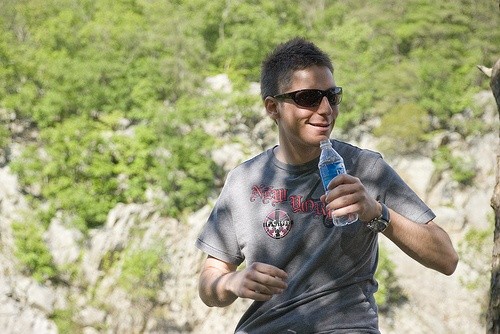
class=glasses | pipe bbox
[274,87,342,108]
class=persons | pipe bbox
[196,37,459,333]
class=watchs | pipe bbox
[367,202,391,234]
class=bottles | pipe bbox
[318,139,358,227]
